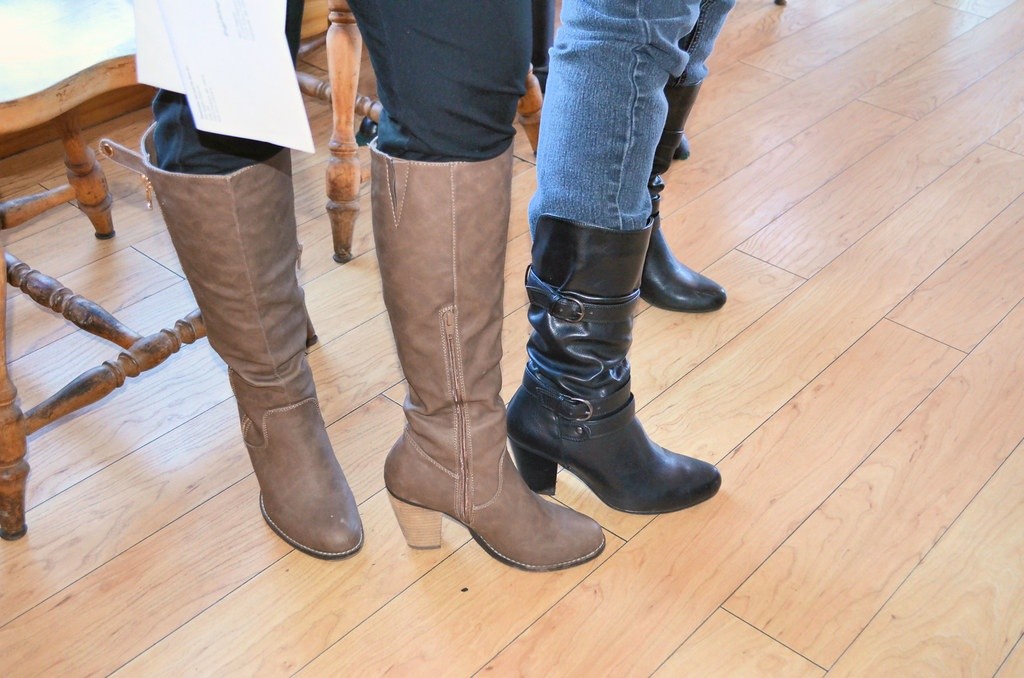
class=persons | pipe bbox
[100,0,604,572]
[507,0,736,515]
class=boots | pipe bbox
[504,213,720,515]
[368,136,608,573]
[97,122,364,561]
[639,78,728,313]
